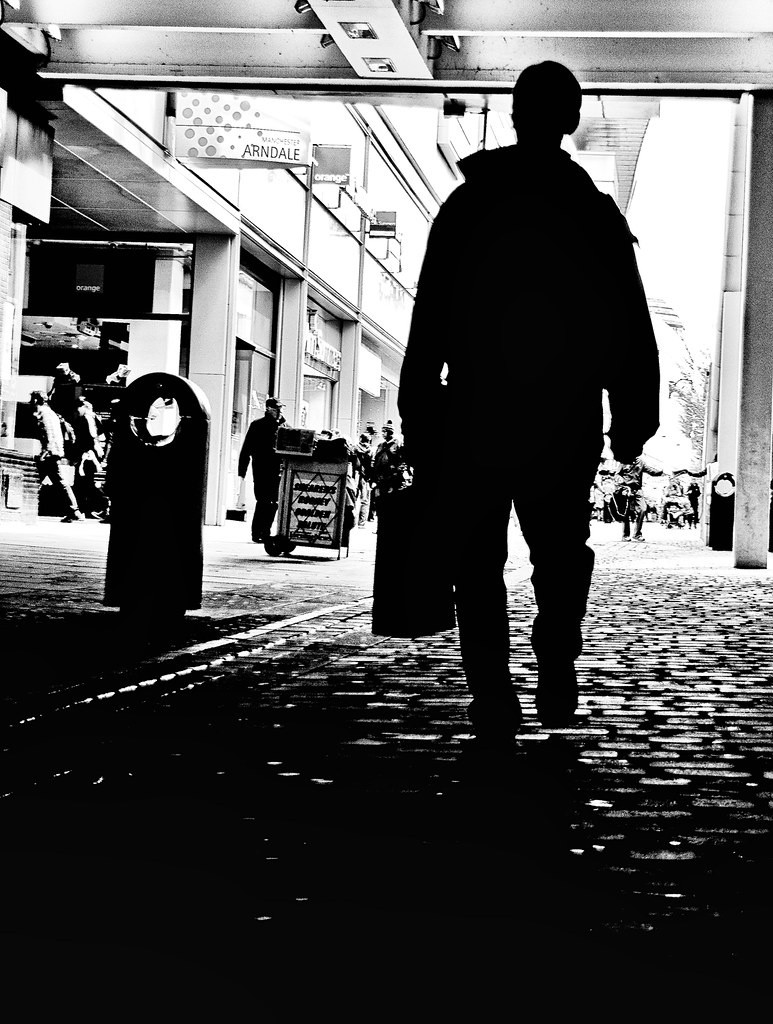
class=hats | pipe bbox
[381,420,393,433]
[265,398,282,411]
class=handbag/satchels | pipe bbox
[372,480,456,639]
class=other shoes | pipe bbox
[634,534,645,541]
[534,656,577,726]
[466,699,516,749]
[623,536,631,541]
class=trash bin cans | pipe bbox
[708,472,736,552]
[98,370,211,612]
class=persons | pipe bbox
[613,455,662,542]
[61,395,120,524]
[317,419,403,529]
[240,397,293,541]
[396,61,662,758]
[688,478,701,529]
[26,391,85,522]
